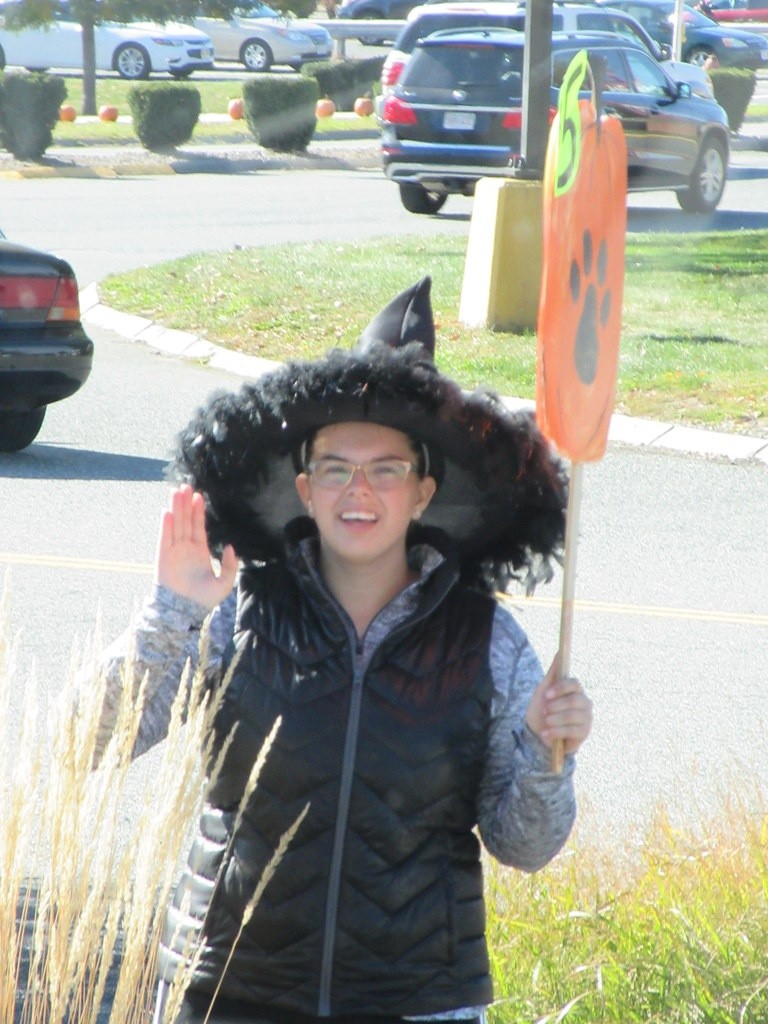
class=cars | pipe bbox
[174,0,334,71]
[0,0,215,80]
[0,231,94,451]
[337,0,460,44]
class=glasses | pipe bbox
[307,460,419,489]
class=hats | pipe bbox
[160,276,568,609]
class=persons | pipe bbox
[58,419,593,1024]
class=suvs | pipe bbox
[338,0,768,213]
